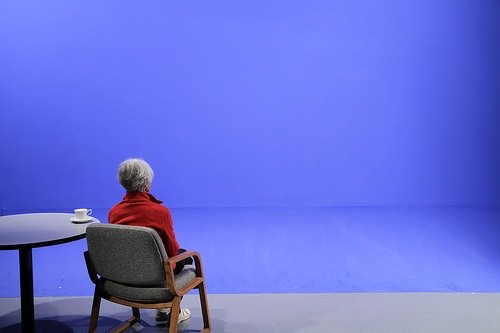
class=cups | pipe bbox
[74,208,92,220]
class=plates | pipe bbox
[70,217,92,222]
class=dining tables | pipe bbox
[0,213,101,333]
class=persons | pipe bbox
[108,159,192,326]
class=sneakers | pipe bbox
[156,305,191,328]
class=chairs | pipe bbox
[83,223,213,333]
